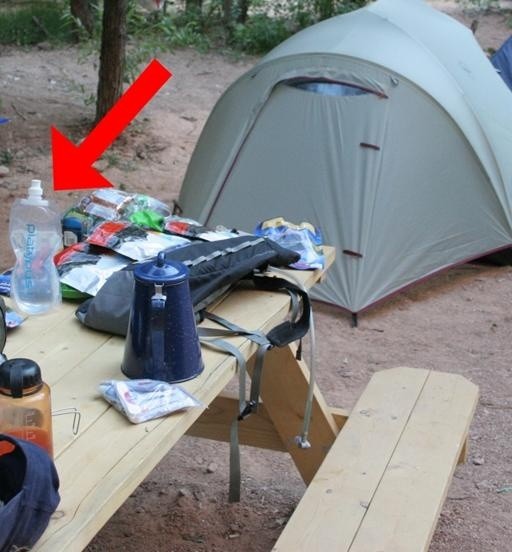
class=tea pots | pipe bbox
[120,252,206,381]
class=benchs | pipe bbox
[275,362,481,551]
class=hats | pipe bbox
[1,433,61,552]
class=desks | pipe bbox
[1,228,348,552]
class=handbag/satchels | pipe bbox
[76,235,302,338]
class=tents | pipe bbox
[176,0,512,327]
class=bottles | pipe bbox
[1,356,53,461]
